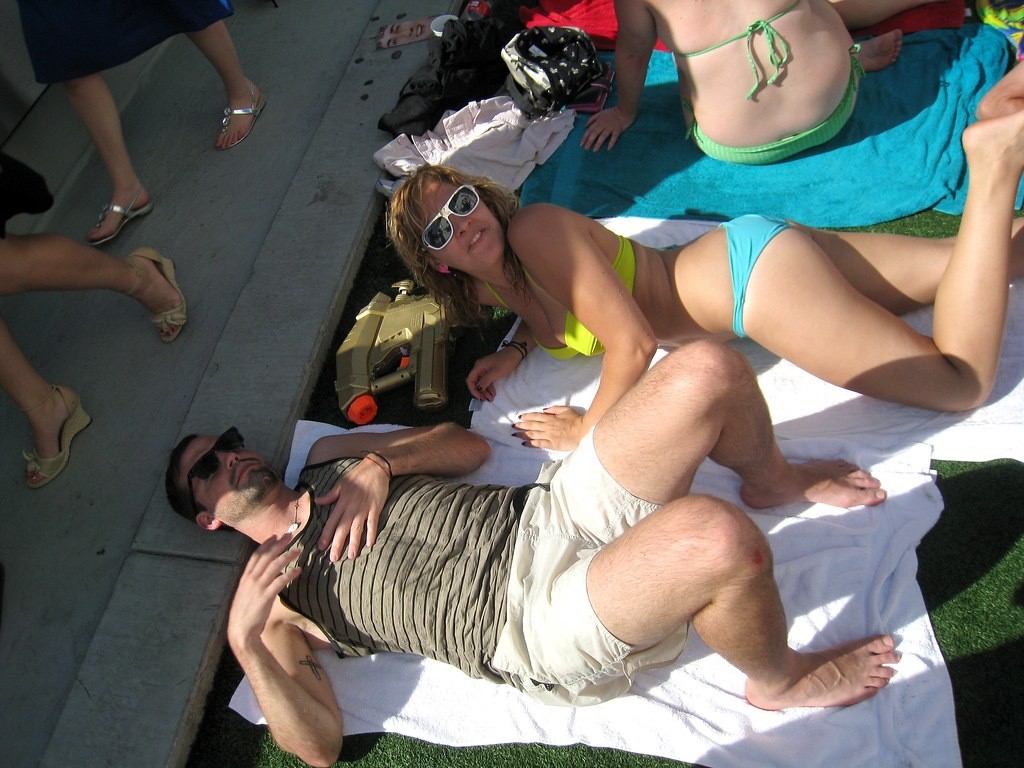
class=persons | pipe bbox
[580,0,902,164]
[976,60,1024,119]
[17,0,267,244]
[164,340,902,768]
[829,0,934,29]
[389,110,1024,451]
[1,148,187,487]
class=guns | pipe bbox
[333,277,479,426]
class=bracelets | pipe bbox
[364,451,394,479]
[501,340,527,360]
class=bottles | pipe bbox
[460,0,495,22]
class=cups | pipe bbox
[431,15,458,37]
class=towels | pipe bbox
[225,416,962,768]
[469,22,1024,232]
[512,0,965,52]
[464,215,1024,465]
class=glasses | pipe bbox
[187,426,244,515]
[422,184,479,252]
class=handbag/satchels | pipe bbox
[500,25,604,121]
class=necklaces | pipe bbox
[287,493,299,533]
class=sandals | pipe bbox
[84,184,154,246]
[20,385,91,489]
[124,248,187,342]
[215,81,267,150]
